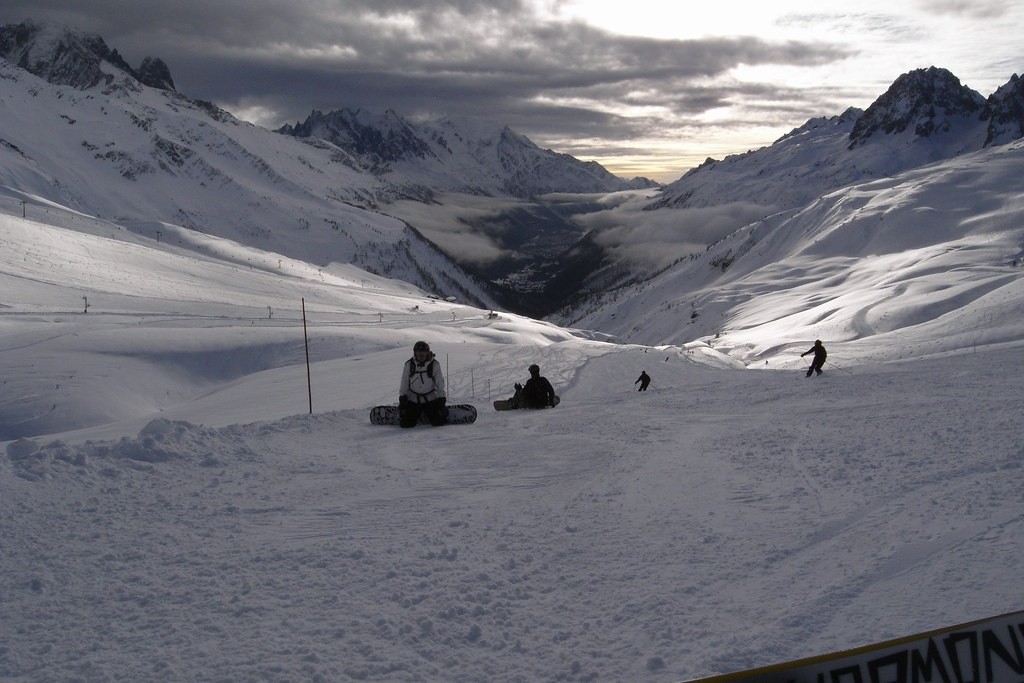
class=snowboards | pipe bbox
[369,404,478,426]
[493,395,561,411]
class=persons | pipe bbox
[508,364,555,409]
[397,341,449,428]
[635,370,650,390]
[801,340,827,378]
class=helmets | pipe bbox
[414,341,431,351]
[529,365,540,373]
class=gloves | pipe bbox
[549,400,555,408]
[438,399,451,418]
[514,383,522,393]
[399,399,410,420]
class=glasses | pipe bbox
[415,352,430,357]
